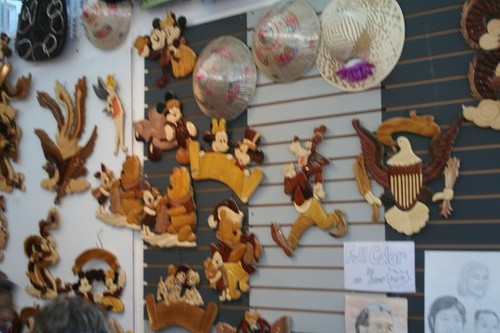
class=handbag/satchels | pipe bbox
[15,0,68,61]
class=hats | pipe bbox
[81,0,133,50]
[193,34,257,122]
[252,0,321,82]
[315,0,406,91]
